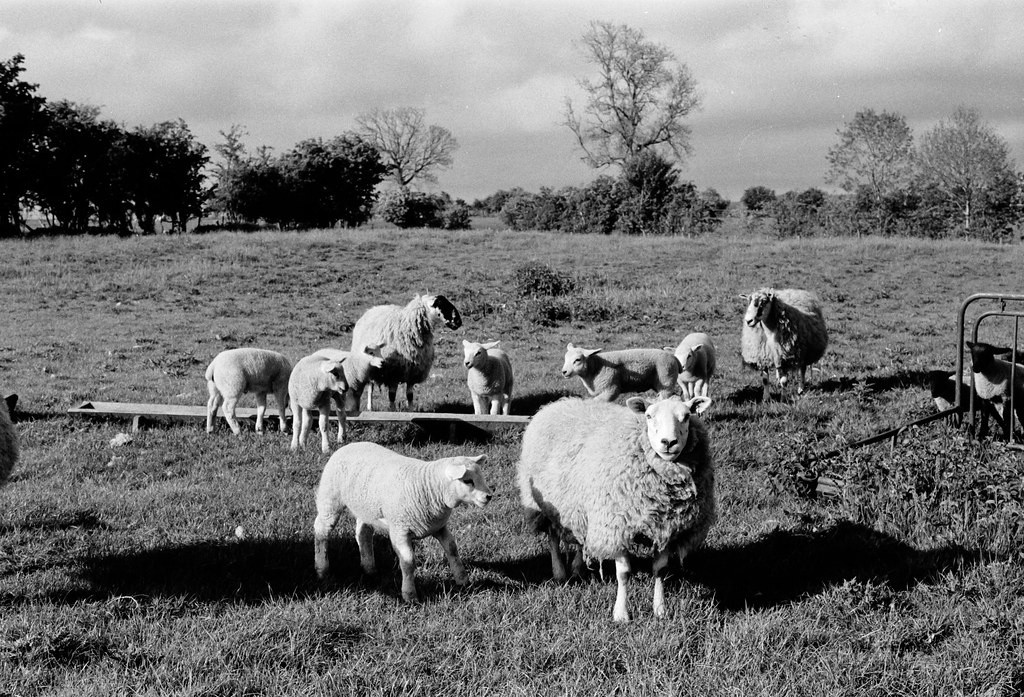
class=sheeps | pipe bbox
[205,348,292,437]
[0,394,20,485]
[518,390,717,623]
[463,340,514,415]
[288,342,388,455]
[314,441,493,604]
[562,343,682,404]
[739,287,828,401]
[663,333,716,396]
[351,287,463,411]
